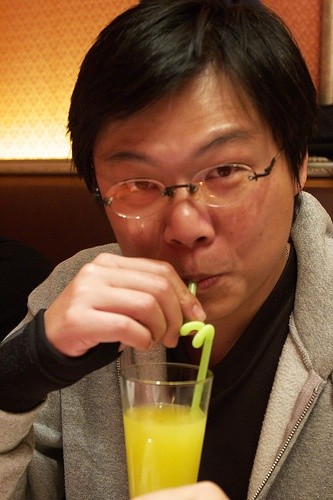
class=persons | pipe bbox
[0,0,333,500]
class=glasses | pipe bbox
[96,144,285,219]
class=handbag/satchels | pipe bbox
[0,235,54,329]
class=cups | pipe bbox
[119,363,214,500]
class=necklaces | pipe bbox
[284,248,289,261]
